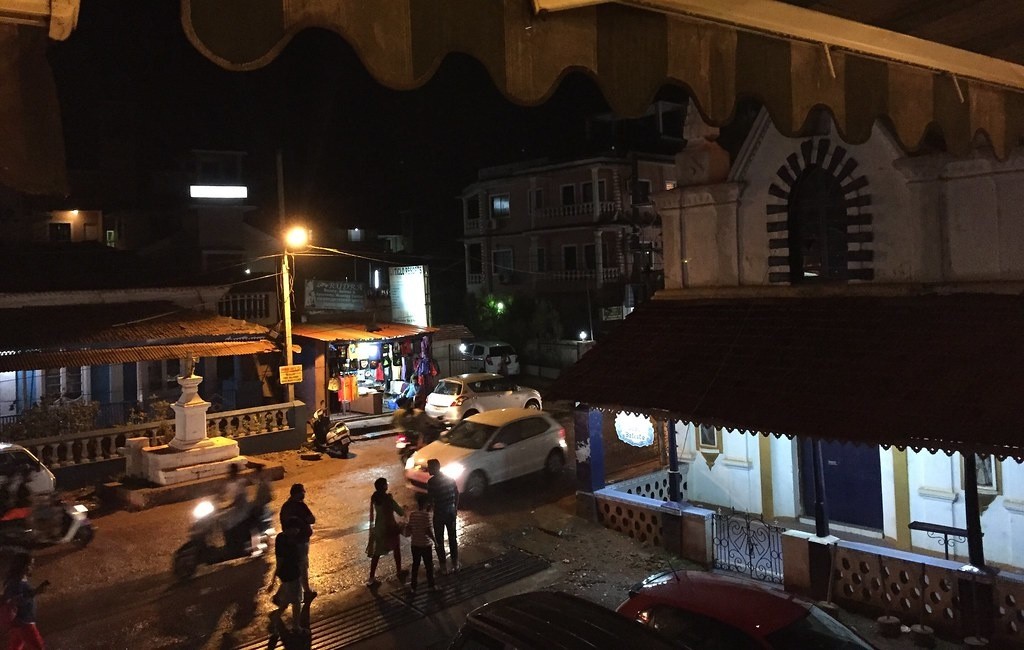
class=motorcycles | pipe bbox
[396,413,451,464]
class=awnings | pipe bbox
[175,0,1024,160]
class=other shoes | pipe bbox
[302,590,317,604]
[438,568,449,575]
[451,561,464,575]
[366,577,381,586]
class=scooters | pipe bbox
[307,402,351,458]
[0,493,94,559]
[170,500,273,572]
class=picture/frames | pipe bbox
[699,423,717,448]
[963,453,997,491]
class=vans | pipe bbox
[0,443,56,497]
[447,590,692,650]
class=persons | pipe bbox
[391,397,444,443]
[485,354,511,379]
[408,492,437,593]
[0,466,48,539]
[211,462,273,546]
[427,459,459,576]
[366,478,409,587]
[271,484,317,636]
[400,376,423,401]
[0,552,51,650]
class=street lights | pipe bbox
[279,221,309,403]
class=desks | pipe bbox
[341,392,382,415]
[906,521,985,561]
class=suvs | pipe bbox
[616,570,878,650]
[403,408,570,503]
[425,373,543,429]
[462,342,520,375]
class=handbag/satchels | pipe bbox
[396,513,411,538]
